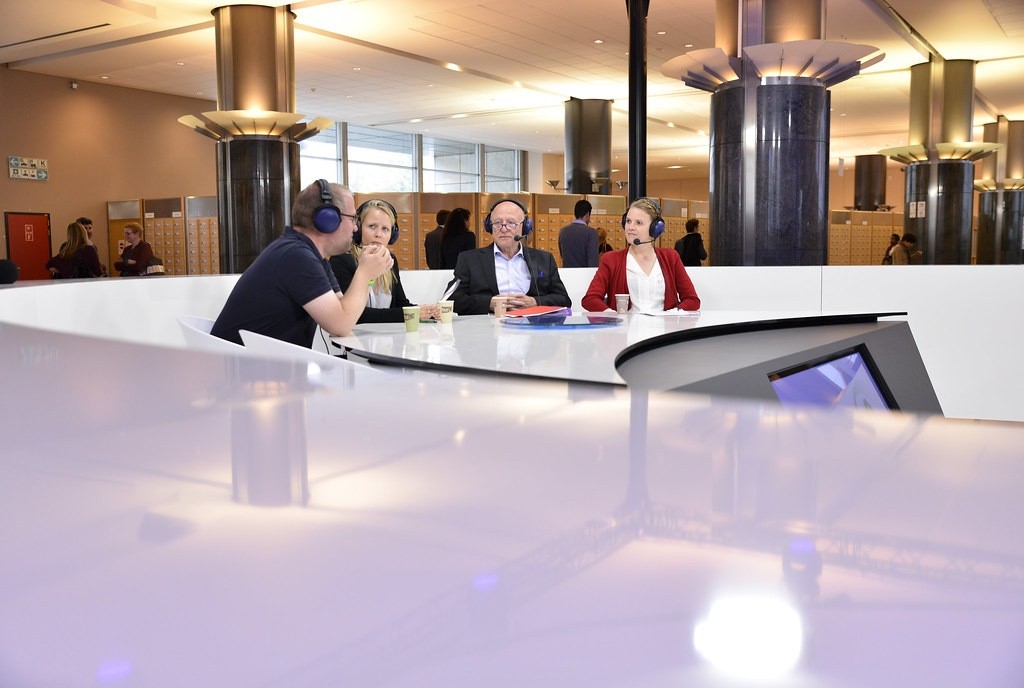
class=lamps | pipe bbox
[973,177,1024,192]
[545,178,629,191]
[878,141,1003,165]
[844,206,854,210]
[177,109,334,141]
[878,205,897,211]
[662,40,885,93]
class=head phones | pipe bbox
[485,198,533,236]
[353,199,400,246]
[621,197,666,239]
[312,179,342,233]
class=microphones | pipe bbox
[514,235,527,241]
[634,238,657,245]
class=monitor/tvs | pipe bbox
[768,344,893,413]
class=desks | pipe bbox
[329,310,907,391]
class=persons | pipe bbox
[674,218,707,266]
[46,218,103,279]
[211,178,394,350]
[558,200,613,269]
[581,199,701,313]
[882,233,922,265]
[114,222,154,277]
[330,199,436,323]
[443,199,572,315]
[425,208,475,270]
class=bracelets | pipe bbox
[368,280,374,285]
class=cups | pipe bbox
[615,294,630,314]
[492,297,507,318]
[437,300,454,323]
[402,306,421,332]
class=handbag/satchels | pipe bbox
[140,257,164,276]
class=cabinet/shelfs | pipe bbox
[828,211,903,265]
[143,197,221,275]
[352,192,714,270]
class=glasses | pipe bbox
[490,222,523,230]
[341,214,359,224]
[124,232,133,235]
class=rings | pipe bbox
[428,309,430,314]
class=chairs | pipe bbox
[179,316,370,369]
